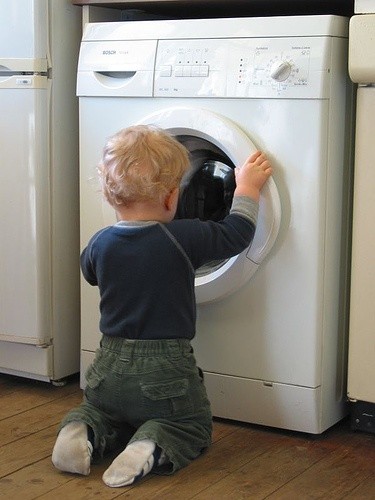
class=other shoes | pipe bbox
[61,449,62,449]
[52,422,94,475]
[102,439,161,485]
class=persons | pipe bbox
[52,126,273,488]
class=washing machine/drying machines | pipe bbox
[76,13,344,434]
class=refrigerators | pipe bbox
[0,0,83,387]
